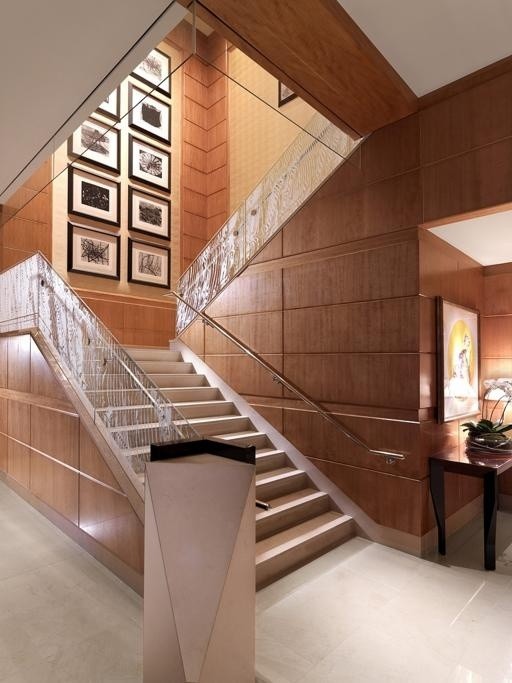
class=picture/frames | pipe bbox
[127,46,171,288]
[278,80,297,107]
[67,78,120,280]
[433,294,482,425]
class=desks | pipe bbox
[428,439,512,571]
